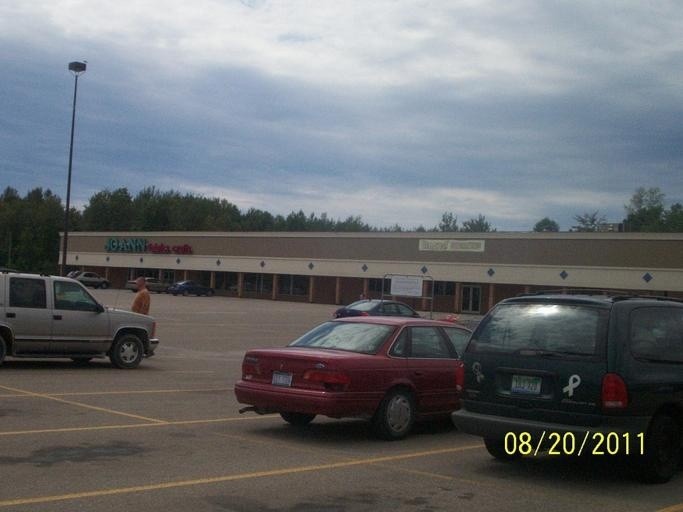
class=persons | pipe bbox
[131,276,155,358]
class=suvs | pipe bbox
[450,288,683,485]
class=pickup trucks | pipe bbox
[0,267,160,370]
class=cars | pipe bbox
[234,316,475,443]
[331,299,421,318]
[66,270,215,297]
